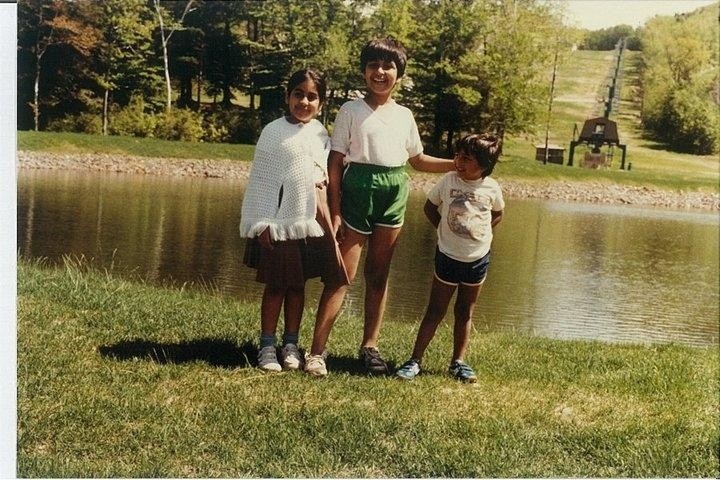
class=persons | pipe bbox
[394,131,507,383]
[237,65,350,373]
[303,35,457,376]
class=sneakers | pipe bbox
[359,346,389,374]
[280,342,303,371]
[303,347,328,376]
[256,343,284,372]
[396,359,422,379]
[449,360,477,384]
